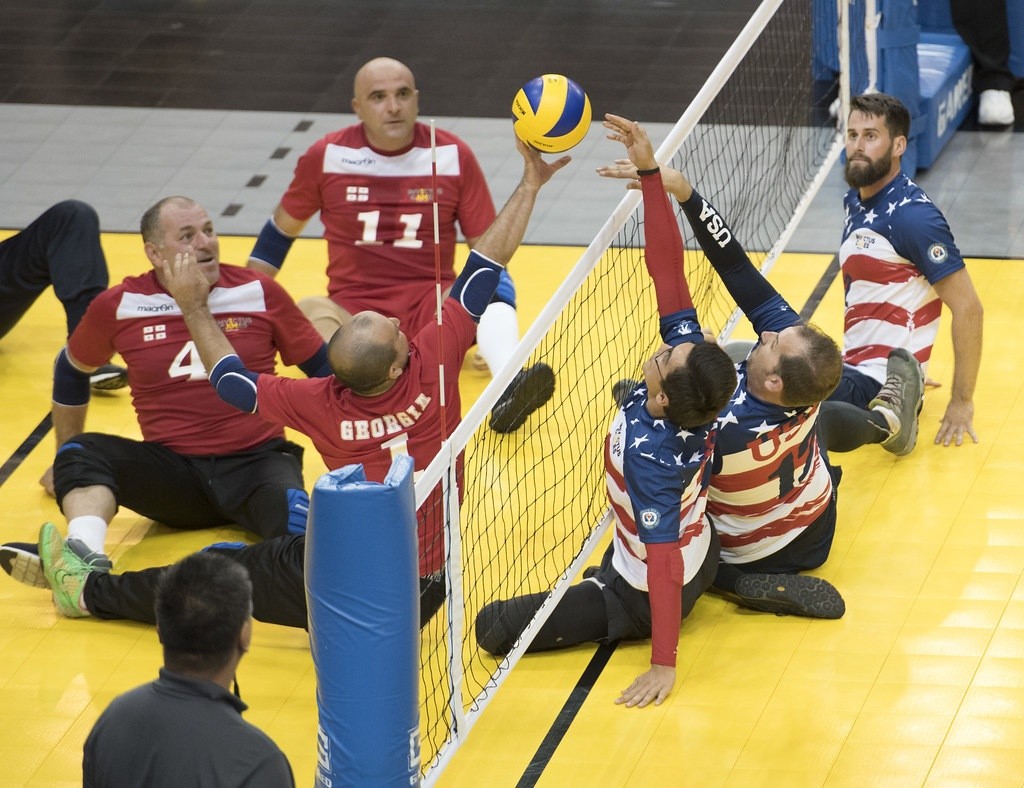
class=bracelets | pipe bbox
[636,167,661,176]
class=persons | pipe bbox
[828,0,1014,124]
[82,551,296,788]
[475,94,984,707]
[0,56,572,632]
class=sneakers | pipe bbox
[612,378,640,409]
[735,572,846,620]
[866,348,924,456]
[0,538,112,589]
[489,362,555,433]
[583,567,601,578]
[89,361,129,389]
[39,522,114,618]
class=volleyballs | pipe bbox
[510,72,592,154]
